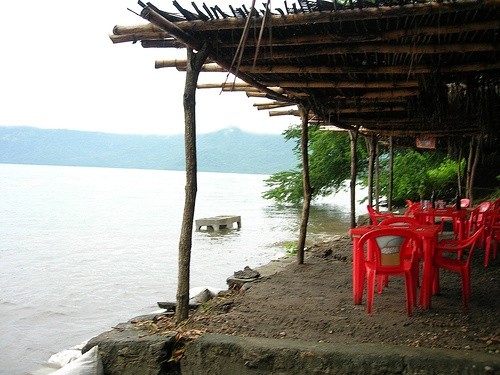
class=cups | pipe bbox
[420,199,429,211]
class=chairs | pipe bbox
[349,198,499,318]
